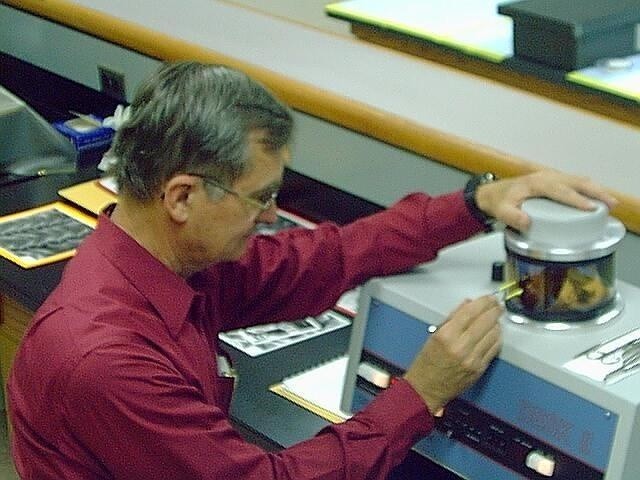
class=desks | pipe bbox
[1,86,418,480]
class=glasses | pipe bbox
[202,173,278,213]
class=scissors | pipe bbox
[587,337,640,365]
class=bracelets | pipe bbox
[463,171,496,226]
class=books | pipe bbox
[279,352,353,420]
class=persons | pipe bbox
[7,59,621,479]
